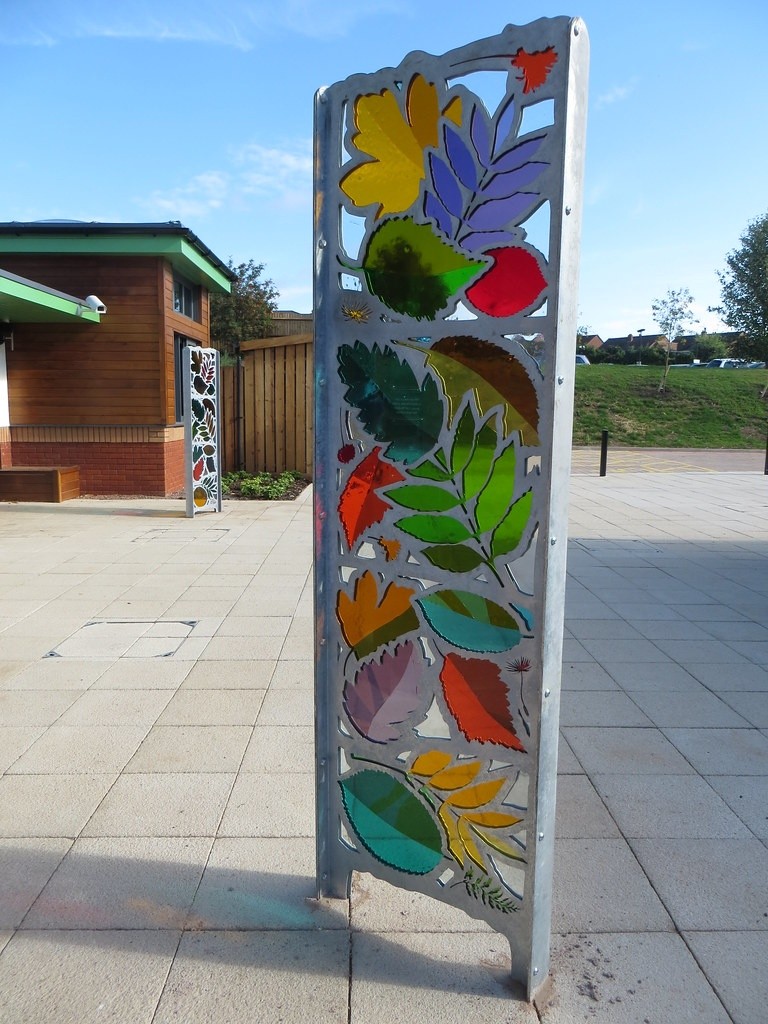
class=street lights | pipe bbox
[637,328,646,366]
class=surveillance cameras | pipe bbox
[86,295,107,313]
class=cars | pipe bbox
[747,361,767,369]
[576,354,591,366]
[690,363,709,368]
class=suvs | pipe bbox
[706,358,748,371]
